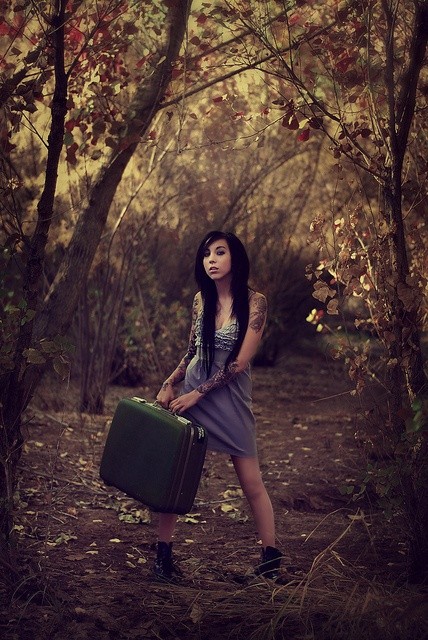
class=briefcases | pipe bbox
[101,398,207,515]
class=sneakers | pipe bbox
[258,546,283,582]
[152,540,181,582]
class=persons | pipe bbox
[156,230,283,584]
